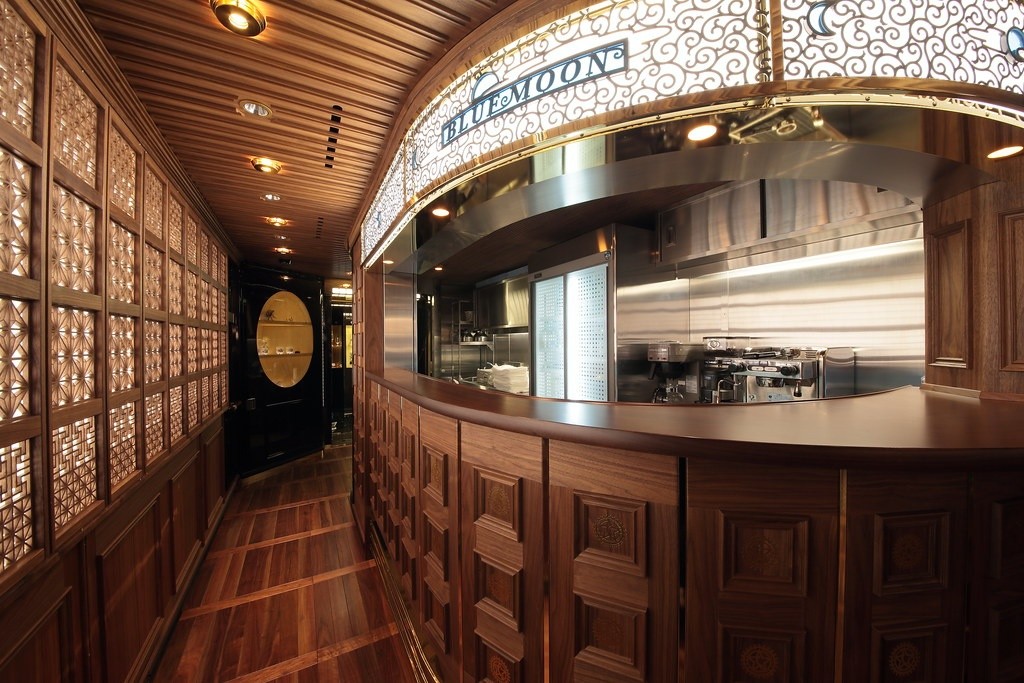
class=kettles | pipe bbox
[461,330,475,342]
[474,328,488,341]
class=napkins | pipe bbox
[493,364,530,393]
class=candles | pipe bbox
[293,368,297,381]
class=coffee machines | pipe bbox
[647,340,707,403]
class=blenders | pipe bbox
[700,333,750,403]
